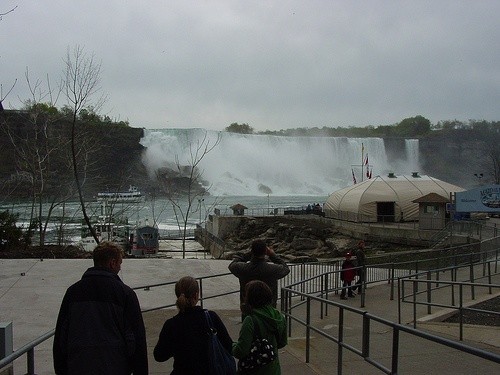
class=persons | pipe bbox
[53,242,148,375]
[352,240,367,294]
[233,280,289,375]
[227,240,290,321]
[153,277,236,375]
[340,252,356,300]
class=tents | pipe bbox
[323,172,469,222]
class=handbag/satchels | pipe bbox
[238,315,276,373]
[203,311,237,375]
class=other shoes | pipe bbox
[351,290,356,295]
[341,296,348,300]
[348,293,355,297]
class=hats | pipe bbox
[249,239,266,250]
[245,280,271,301]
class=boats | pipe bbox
[79,221,137,253]
[96,184,145,204]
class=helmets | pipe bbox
[346,253,351,256]
